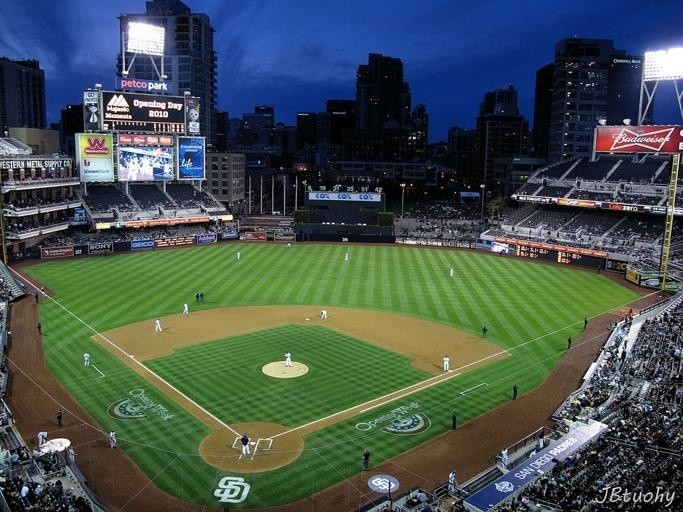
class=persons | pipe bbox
[1,155,681,510]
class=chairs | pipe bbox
[44,177,237,245]
[0,261,103,510]
[394,151,683,510]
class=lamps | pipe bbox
[638,47,682,124]
[119,16,168,82]
